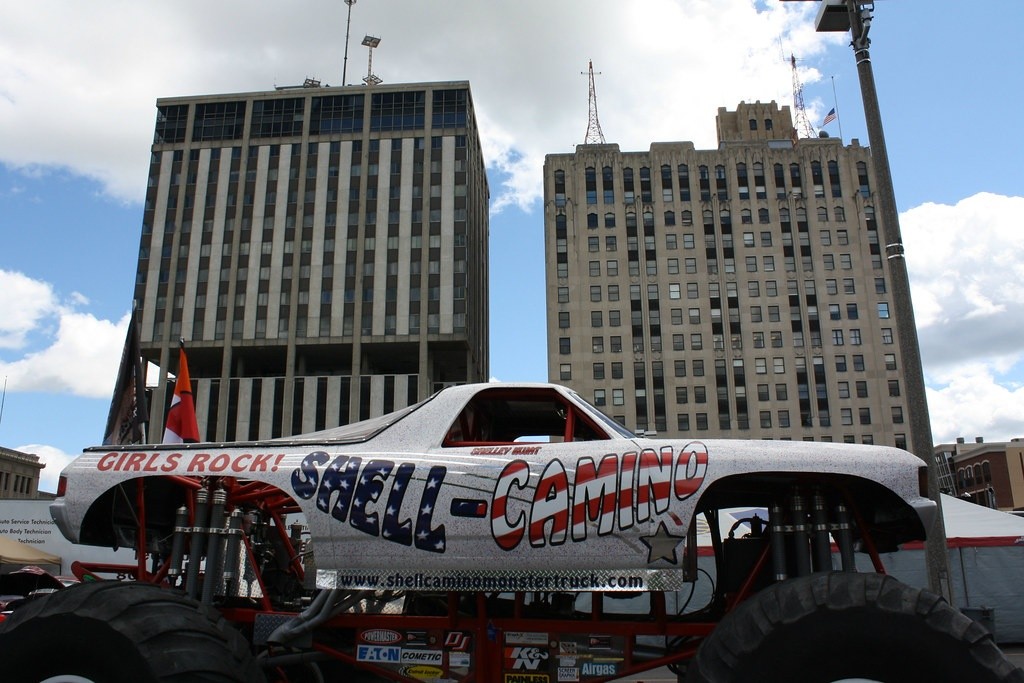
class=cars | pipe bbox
[0,566,79,619]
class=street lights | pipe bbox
[814,0,951,598]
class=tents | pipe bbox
[487,489,1024,656]
[0,534,62,576]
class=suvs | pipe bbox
[1,380,1023,683]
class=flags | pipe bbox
[823,108,835,126]
[102,306,149,445]
[162,348,200,444]
